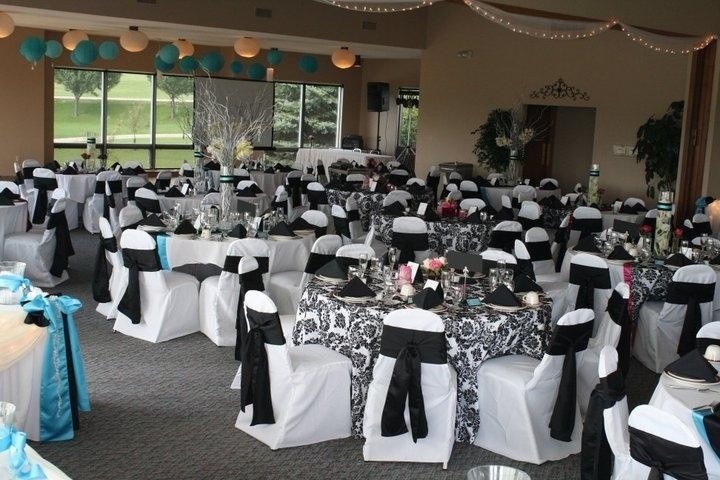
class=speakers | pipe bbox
[366,81,391,112]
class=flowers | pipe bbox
[206,137,254,165]
[495,124,535,149]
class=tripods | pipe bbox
[396,108,416,165]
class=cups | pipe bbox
[0,260,26,306]
[523,291,539,304]
[0,400,19,479]
[466,464,533,480]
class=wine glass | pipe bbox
[154,197,286,241]
[588,226,718,268]
[346,242,517,312]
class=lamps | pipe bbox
[0,12,16,39]
[62,28,89,51]
[171,38,195,59]
[396,91,419,109]
[331,48,356,68]
[120,26,149,52]
[234,36,262,57]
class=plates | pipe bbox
[660,360,720,388]
[314,270,374,305]
[269,228,316,240]
[482,299,530,312]
